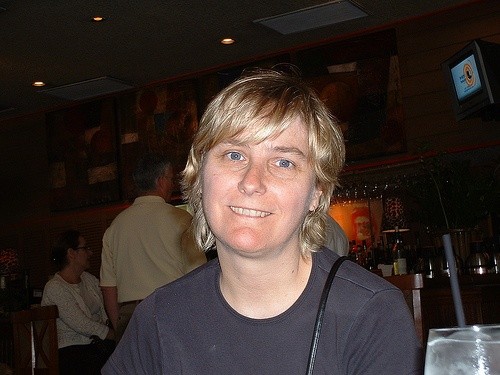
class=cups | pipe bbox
[424,325,500,375]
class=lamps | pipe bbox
[380,194,413,276]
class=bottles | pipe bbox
[343,225,443,276]
[455,241,500,273]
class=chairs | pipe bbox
[9,306,61,375]
[383,271,424,355]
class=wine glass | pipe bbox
[327,172,427,205]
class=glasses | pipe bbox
[76,245,91,251]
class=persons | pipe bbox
[99,154,208,340]
[322,213,349,258]
[100,70,423,374]
[43,231,120,375]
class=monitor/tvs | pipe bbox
[444,39,500,118]
